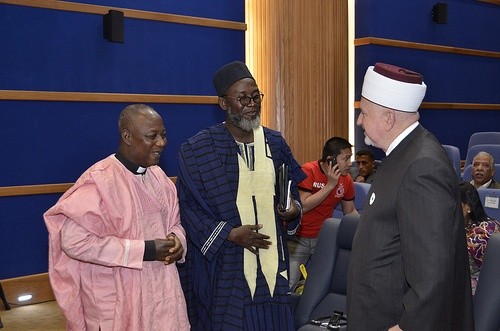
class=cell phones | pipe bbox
[327,155,337,171]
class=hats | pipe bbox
[361,63,428,113]
[213,62,256,95]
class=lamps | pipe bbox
[432,3,448,24]
[103,9,125,43]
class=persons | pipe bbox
[284,137,361,289]
[60,104,188,331]
[178,75,303,331]
[346,63,464,331]
[459,182,500,295]
[352,149,378,184]
[469,151,500,189]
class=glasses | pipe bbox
[223,92,264,105]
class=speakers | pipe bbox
[103,9,124,44]
[432,3,448,24]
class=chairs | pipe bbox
[289,132,500,331]
